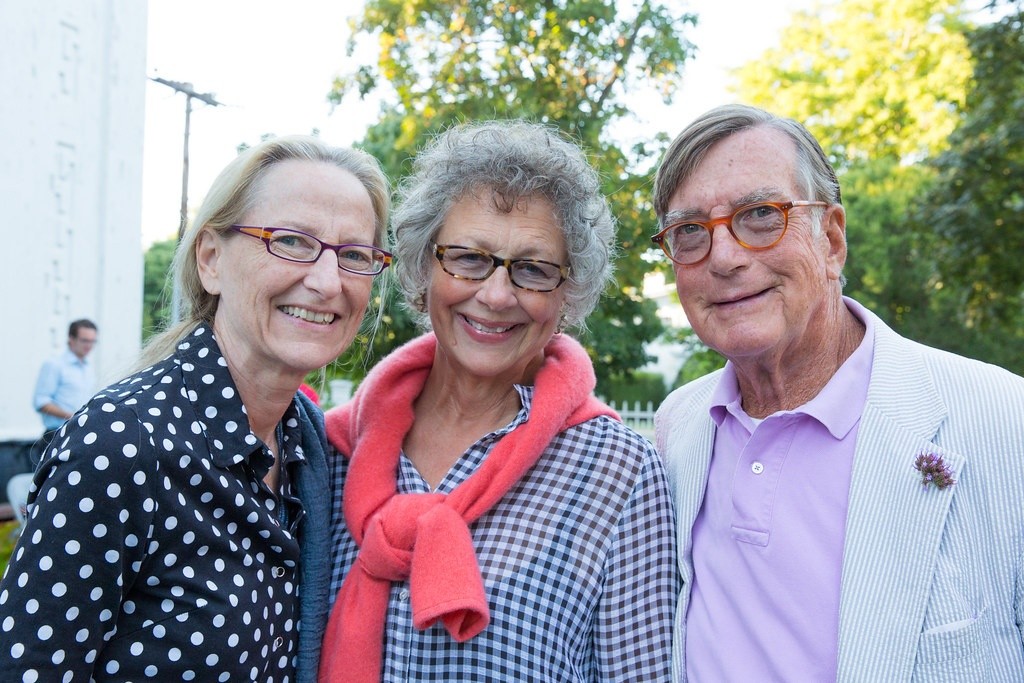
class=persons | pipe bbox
[32,319,99,458]
[319,121,683,683]
[650,103,1023,683]
[0,136,394,683]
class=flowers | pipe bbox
[912,451,956,488]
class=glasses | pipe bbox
[229,223,393,275]
[428,240,571,293]
[651,200,828,266]
